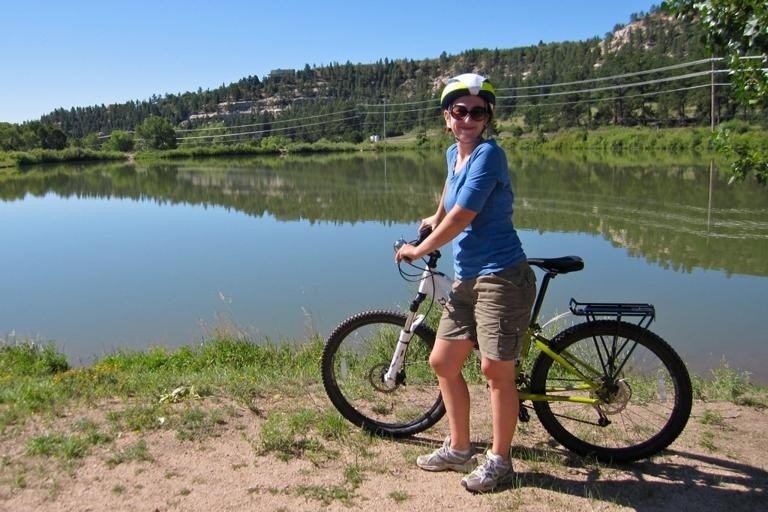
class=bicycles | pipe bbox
[320,225,694,464]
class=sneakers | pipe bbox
[461,448,515,492]
[416,435,478,474]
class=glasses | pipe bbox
[449,105,490,121]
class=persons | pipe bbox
[394,72,536,493]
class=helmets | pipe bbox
[440,73,496,108]
